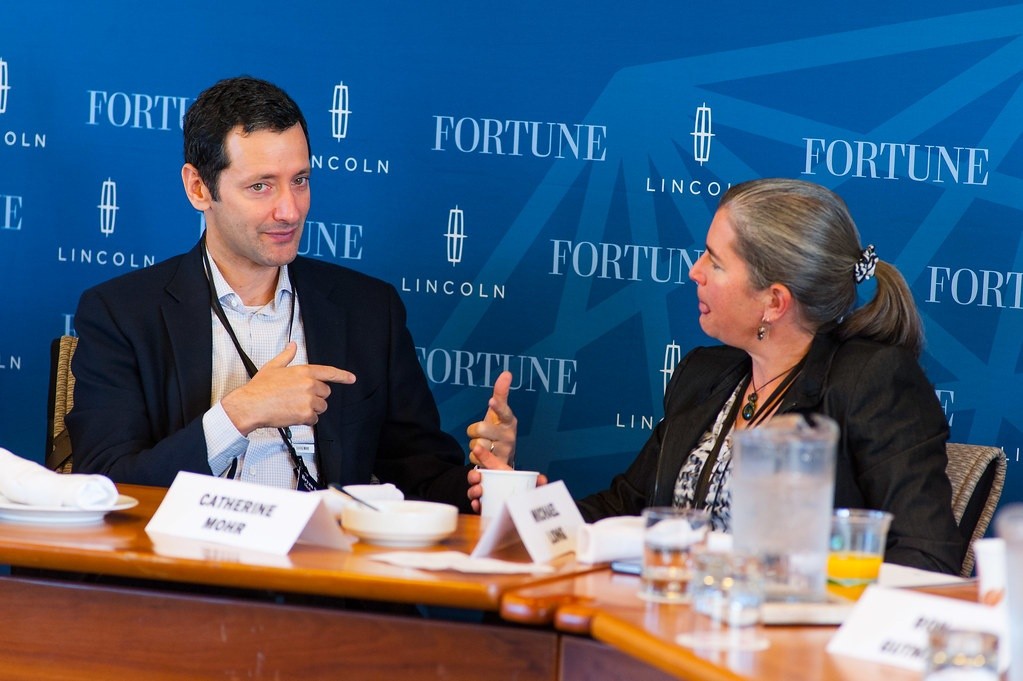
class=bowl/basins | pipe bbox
[343,500,458,549]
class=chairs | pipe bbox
[945,444,1008,577]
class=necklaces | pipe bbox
[742,363,797,421]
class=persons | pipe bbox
[469,177,963,577]
[62,72,516,514]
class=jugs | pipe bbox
[732,413,840,609]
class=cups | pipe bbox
[972,538,1005,607]
[996,504,1023,681]
[641,507,715,604]
[827,509,894,603]
[477,468,539,545]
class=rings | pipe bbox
[490,441,494,452]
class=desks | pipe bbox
[0,478,1008,681]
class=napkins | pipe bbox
[0,446,118,507]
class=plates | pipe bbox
[597,515,644,528]
[0,494,140,525]
[310,484,405,520]
